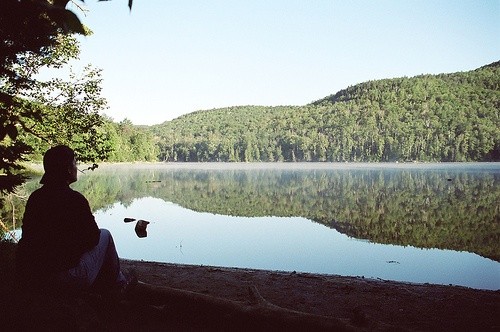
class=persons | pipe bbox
[16,145,127,296]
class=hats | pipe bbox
[39,145,76,185]
